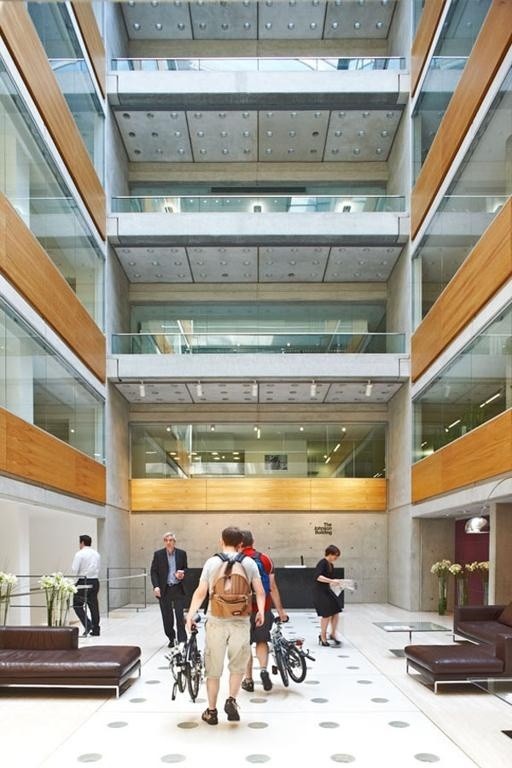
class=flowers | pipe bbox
[464,559,494,593]
[38,572,78,627]
[0,570,17,624]
[448,563,467,606]
[431,558,452,615]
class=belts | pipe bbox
[167,582,182,587]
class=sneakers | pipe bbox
[202,671,272,725]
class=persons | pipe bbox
[238,529,287,692]
[185,525,266,725]
[311,544,343,646]
[64,534,100,636]
[150,532,188,647]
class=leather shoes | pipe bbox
[168,641,174,647]
[83,625,94,635]
[90,632,100,636]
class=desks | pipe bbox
[372,620,452,658]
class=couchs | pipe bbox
[0,625,143,699]
[402,603,512,693]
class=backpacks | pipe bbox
[211,553,252,618]
[252,552,269,603]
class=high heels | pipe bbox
[319,635,340,646]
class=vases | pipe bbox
[482,582,488,606]
[437,577,449,616]
[456,578,468,607]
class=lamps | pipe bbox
[462,473,511,535]
[138,378,375,398]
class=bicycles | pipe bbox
[166,614,205,704]
[268,613,316,688]
